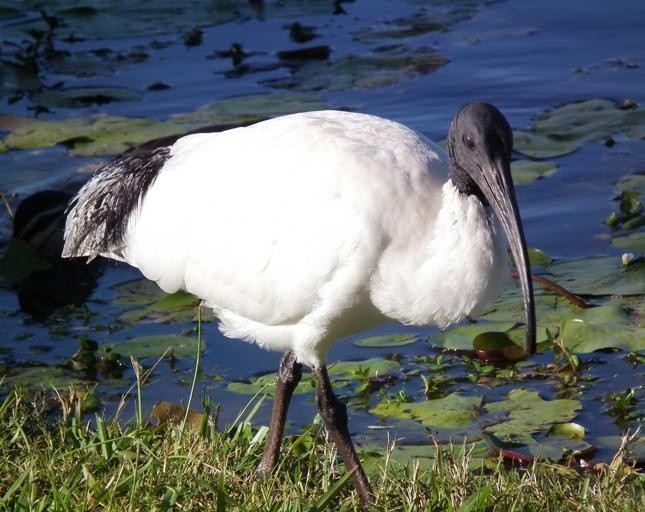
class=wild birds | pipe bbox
[10,100,537,507]
